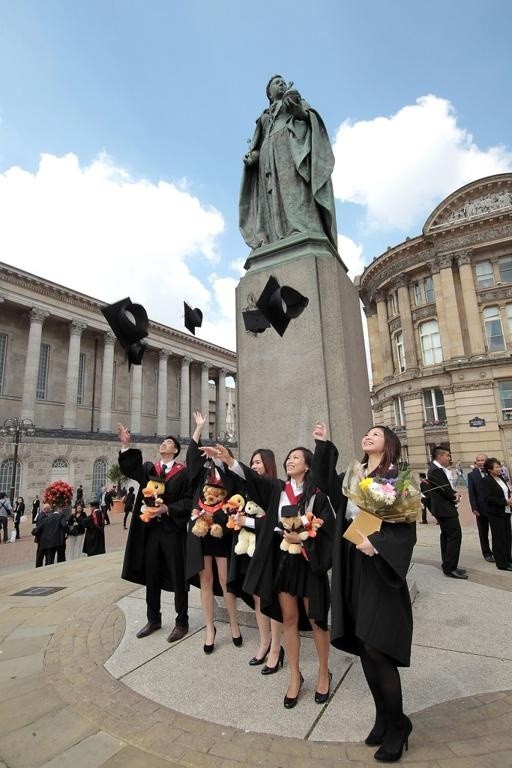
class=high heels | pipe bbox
[248,637,274,666]
[372,713,414,762]
[260,643,285,675]
[230,623,243,647]
[365,713,386,748]
[202,626,217,655]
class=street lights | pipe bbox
[1,417,35,511]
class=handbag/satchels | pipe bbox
[6,509,12,516]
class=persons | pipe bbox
[419,446,512,579]
[115,420,189,643]
[198,446,285,675]
[236,72,339,251]
[213,442,336,709]
[311,418,418,763]
[185,411,243,654]
[0,483,136,568]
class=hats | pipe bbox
[182,300,204,336]
[88,500,100,507]
[100,297,152,348]
[241,308,271,336]
[124,344,147,372]
[255,275,310,339]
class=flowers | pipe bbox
[43,480,75,509]
[357,467,412,519]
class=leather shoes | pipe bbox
[136,622,162,638]
[484,555,495,563]
[498,562,512,571]
[445,570,469,580]
[167,623,189,642]
[455,567,466,575]
[314,672,333,704]
[283,676,304,709]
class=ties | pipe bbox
[159,464,167,480]
[482,470,487,477]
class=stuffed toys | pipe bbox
[274,505,309,555]
[222,493,246,531]
[190,481,228,540]
[138,476,167,525]
[234,500,266,559]
